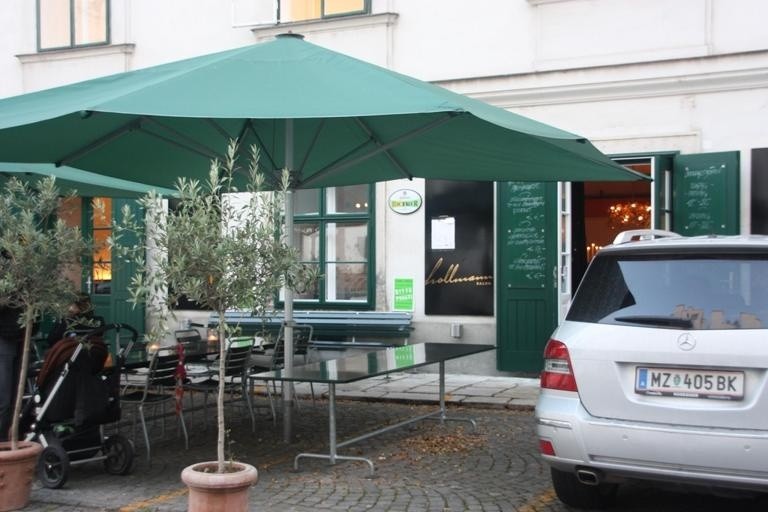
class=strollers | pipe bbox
[23,323,137,490]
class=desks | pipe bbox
[248,341,497,480]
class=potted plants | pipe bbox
[107,136,329,512]
[0,173,108,512]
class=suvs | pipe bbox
[535,225,767,501]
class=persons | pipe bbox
[0,247,33,441]
[45,288,105,351]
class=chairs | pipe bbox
[209,311,415,379]
[120,324,318,467]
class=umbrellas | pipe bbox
[1,26,658,399]
[1,161,194,231]
